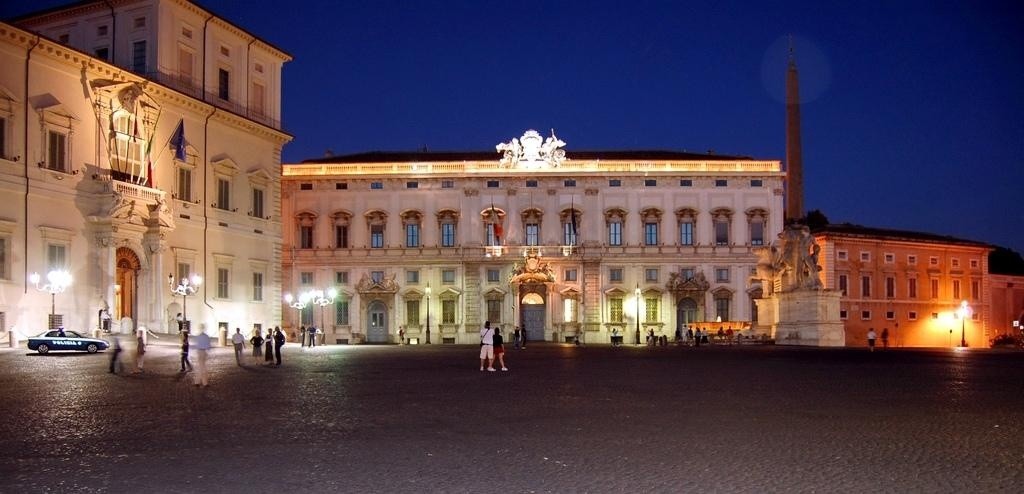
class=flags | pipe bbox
[146,147,154,187]
[571,202,576,234]
[490,205,504,236]
[166,116,186,162]
[132,104,144,141]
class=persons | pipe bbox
[249,329,264,367]
[231,327,245,366]
[190,323,211,388]
[100,309,110,331]
[490,326,508,371]
[398,326,404,345]
[271,326,285,366]
[106,337,122,374]
[880,326,889,347]
[513,326,523,350]
[264,328,275,367]
[300,321,305,347]
[646,328,653,345]
[672,323,734,349]
[574,335,579,345]
[866,326,877,351]
[175,312,182,335]
[135,336,146,373]
[478,319,496,371]
[306,322,315,346]
[180,331,192,372]
[894,321,903,347]
[520,324,526,349]
[611,326,619,345]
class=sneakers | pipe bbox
[487,367,497,371]
[480,367,484,371]
[502,368,508,371]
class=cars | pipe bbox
[26,326,110,354]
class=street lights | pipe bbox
[955,299,973,346]
[634,282,641,343]
[424,281,432,343]
[167,272,203,347]
[309,287,337,345]
[31,268,74,329]
[285,292,312,342]
